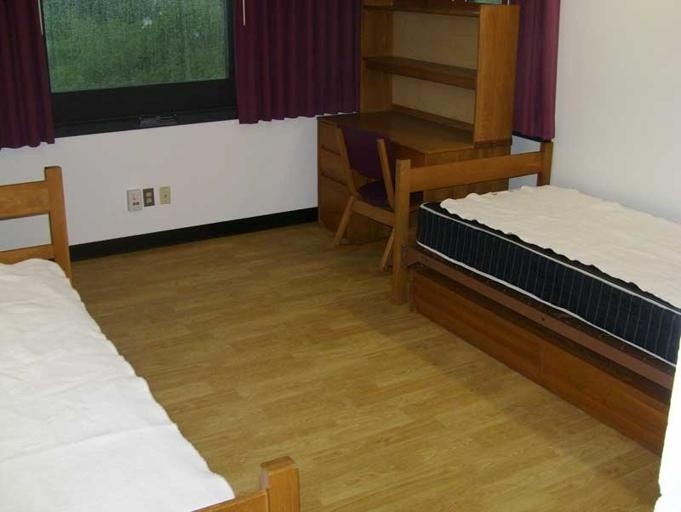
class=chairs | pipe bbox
[327,123,420,272]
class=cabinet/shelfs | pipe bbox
[316,0,520,274]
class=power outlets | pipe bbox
[144,186,154,208]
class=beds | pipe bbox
[0,166,303,512]
[388,140,681,458]
